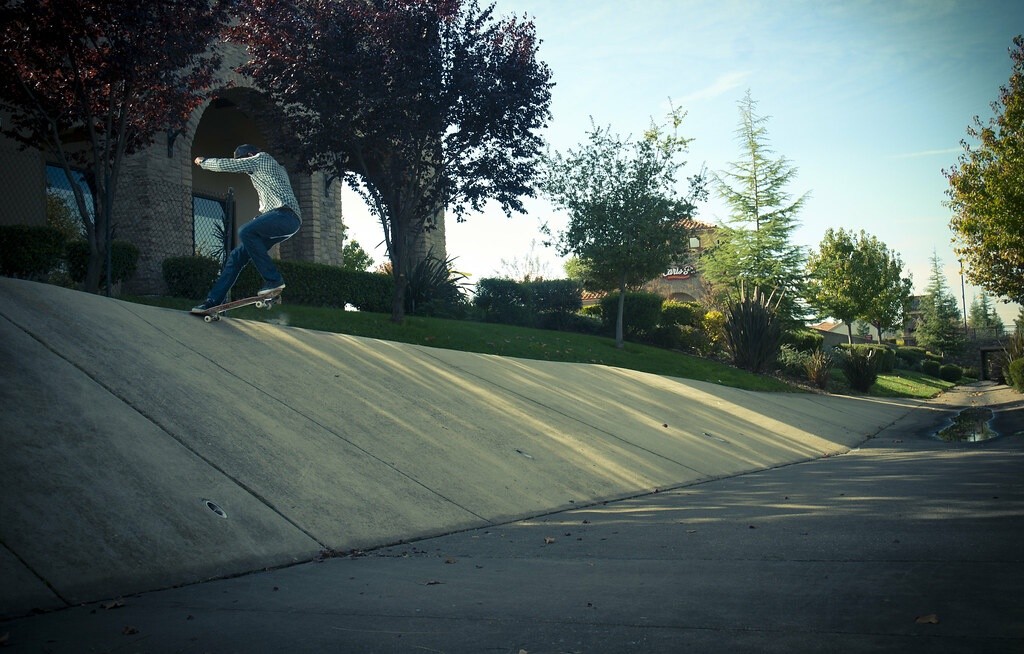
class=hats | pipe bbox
[234,144,256,159]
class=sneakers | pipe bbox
[258,276,286,296]
[192,296,227,313]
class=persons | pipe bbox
[192,143,303,311]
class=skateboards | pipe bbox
[192,288,284,323]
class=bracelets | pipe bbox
[201,158,207,163]
